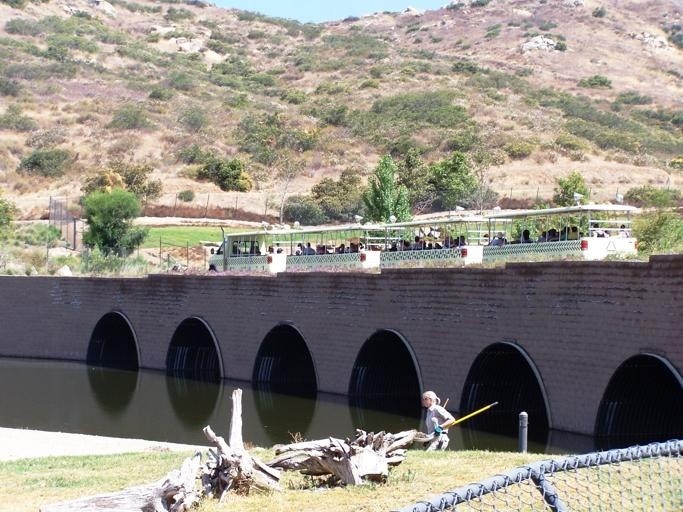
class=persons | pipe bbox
[295,243,303,256]
[521,229,533,243]
[401,242,412,251]
[388,244,397,252]
[334,241,364,254]
[425,235,465,249]
[618,224,630,238]
[411,236,421,250]
[420,389,456,452]
[317,245,330,255]
[538,225,580,242]
[491,232,503,246]
[592,224,609,238]
[302,242,314,255]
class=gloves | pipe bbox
[434,426,443,436]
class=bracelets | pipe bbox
[437,426,443,430]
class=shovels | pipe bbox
[413,402,499,442]
